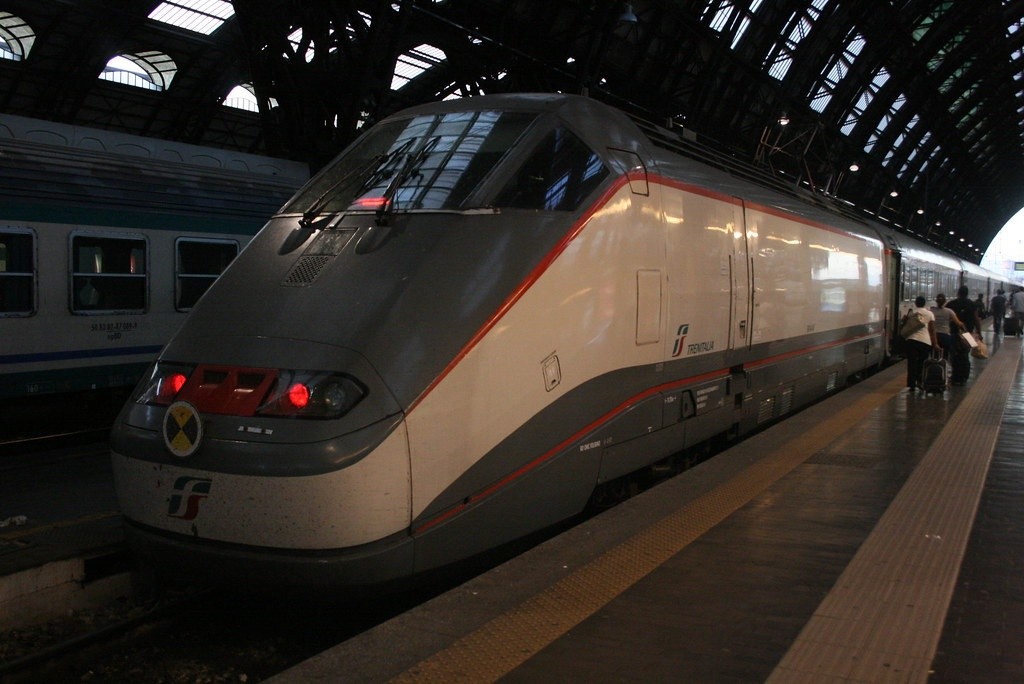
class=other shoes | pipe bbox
[909,387,915,392]
[919,389,924,393]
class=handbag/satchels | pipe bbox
[900,308,926,339]
[971,336,988,359]
[958,326,977,349]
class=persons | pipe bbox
[0,280,34,312]
[905,297,941,392]
[974,293,985,319]
[92,277,135,306]
[179,278,208,308]
[1002,289,1019,318]
[502,175,538,209]
[930,294,966,358]
[991,289,1006,334]
[945,286,983,386]
[1011,287,1024,336]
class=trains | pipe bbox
[0,109,327,403]
[103,90,1024,584]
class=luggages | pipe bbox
[952,349,970,385]
[920,348,946,397]
[1004,311,1016,336]
